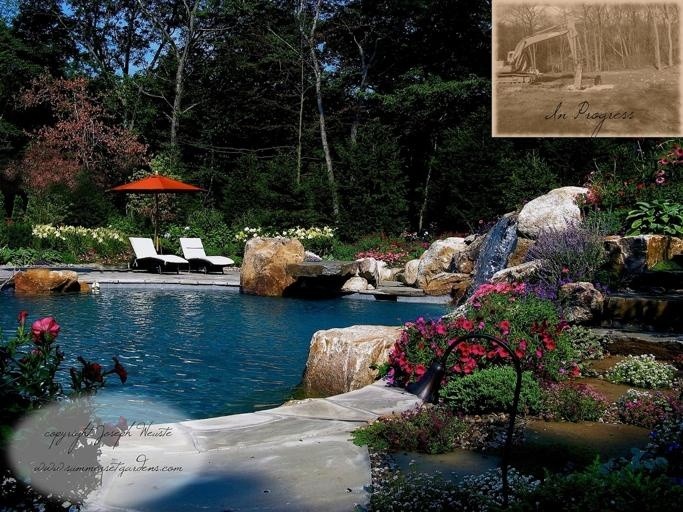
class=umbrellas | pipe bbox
[105,171,207,256]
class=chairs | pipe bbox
[179,235,234,274]
[128,236,195,273]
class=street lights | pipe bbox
[407,333,521,509]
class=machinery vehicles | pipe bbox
[498,20,584,89]
